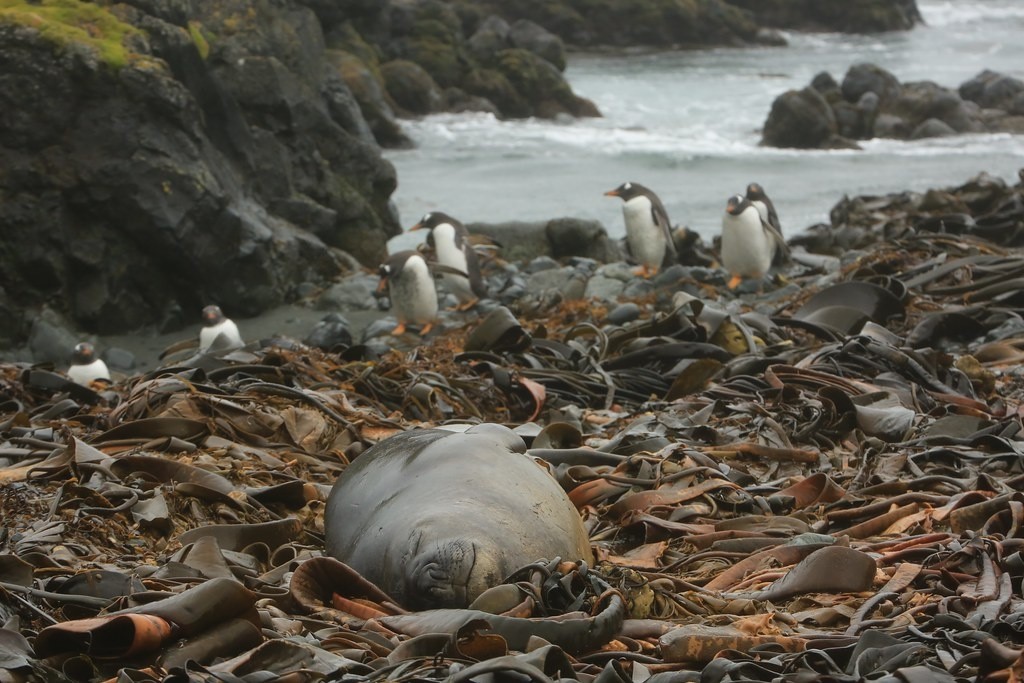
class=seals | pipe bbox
[325,422,593,609]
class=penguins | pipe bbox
[746,183,792,266]
[377,249,438,336]
[198,305,246,353]
[407,211,488,311]
[719,194,783,294]
[68,342,110,388]
[604,182,679,278]
